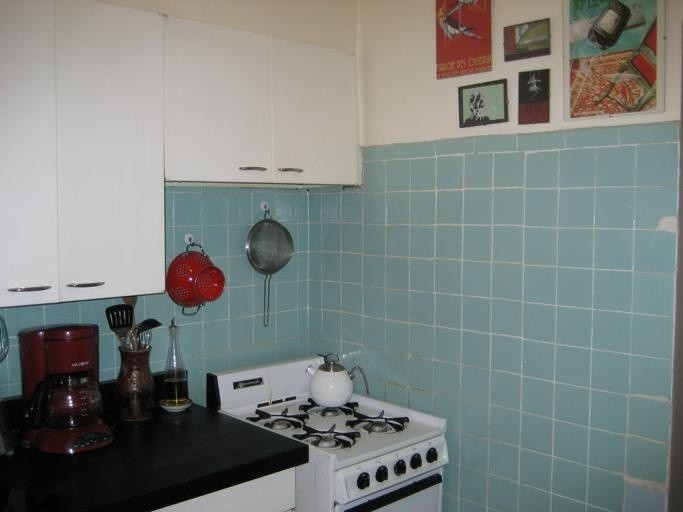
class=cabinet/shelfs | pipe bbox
[457,80,510,128]
[3,2,168,308]
[152,467,296,511]
[166,19,365,187]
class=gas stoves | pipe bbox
[208,356,450,505]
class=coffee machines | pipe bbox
[18,324,115,454]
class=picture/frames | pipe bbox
[563,0,664,124]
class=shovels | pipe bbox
[105,304,133,349]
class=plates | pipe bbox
[160,402,191,412]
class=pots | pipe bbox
[165,243,226,316]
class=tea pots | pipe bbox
[305,354,368,408]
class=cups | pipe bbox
[114,346,155,422]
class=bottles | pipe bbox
[162,326,189,405]
